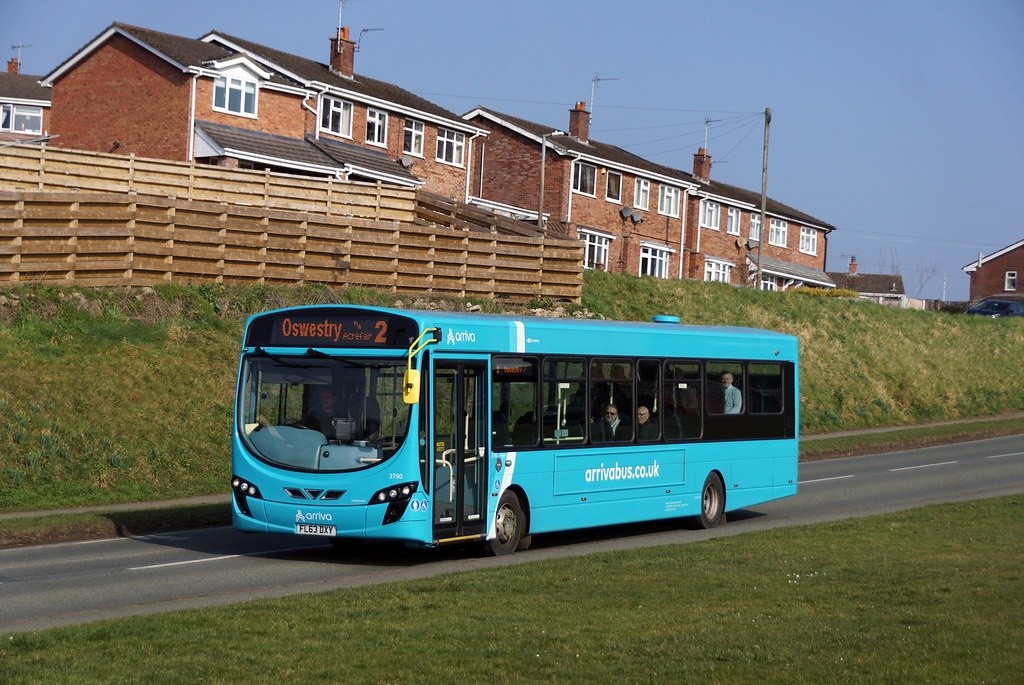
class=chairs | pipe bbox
[347,364,771,444]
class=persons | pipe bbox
[718,372,742,413]
[637,405,658,439]
[291,385,358,441]
[573,365,687,419]
[590,403,629,442]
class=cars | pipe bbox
[964,301,1024,319]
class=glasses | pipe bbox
[605,412,616,415]
[637,413,648,415]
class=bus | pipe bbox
[228,302,801,558]
